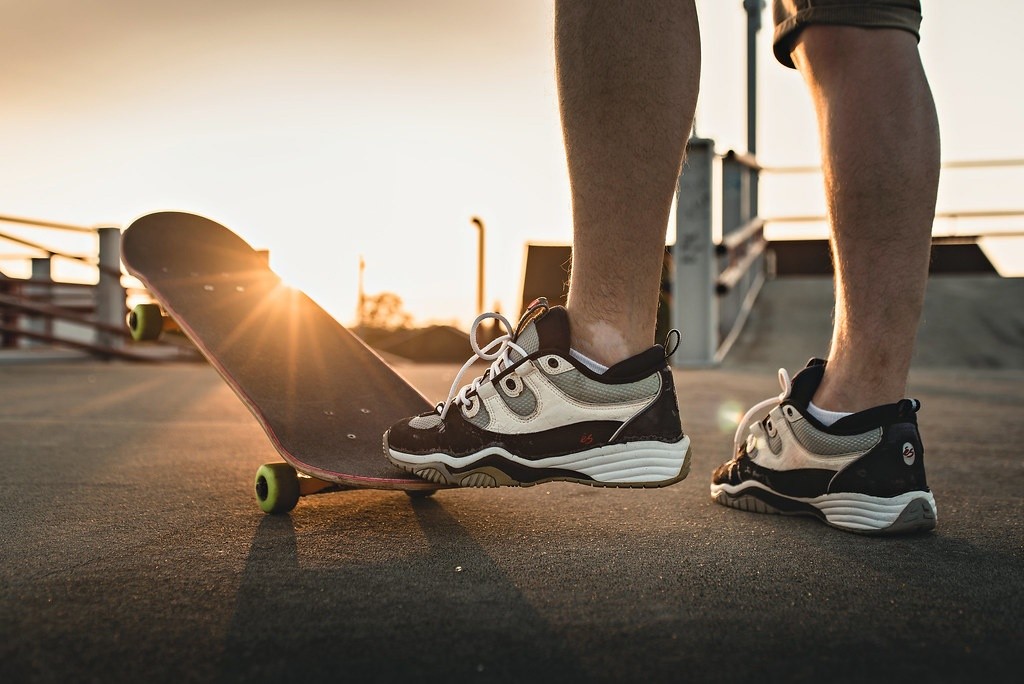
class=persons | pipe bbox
[383,0,940,535]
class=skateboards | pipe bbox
[118,209,467,515]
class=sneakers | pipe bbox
[710,357,939,534]
[382,298,691,489]
